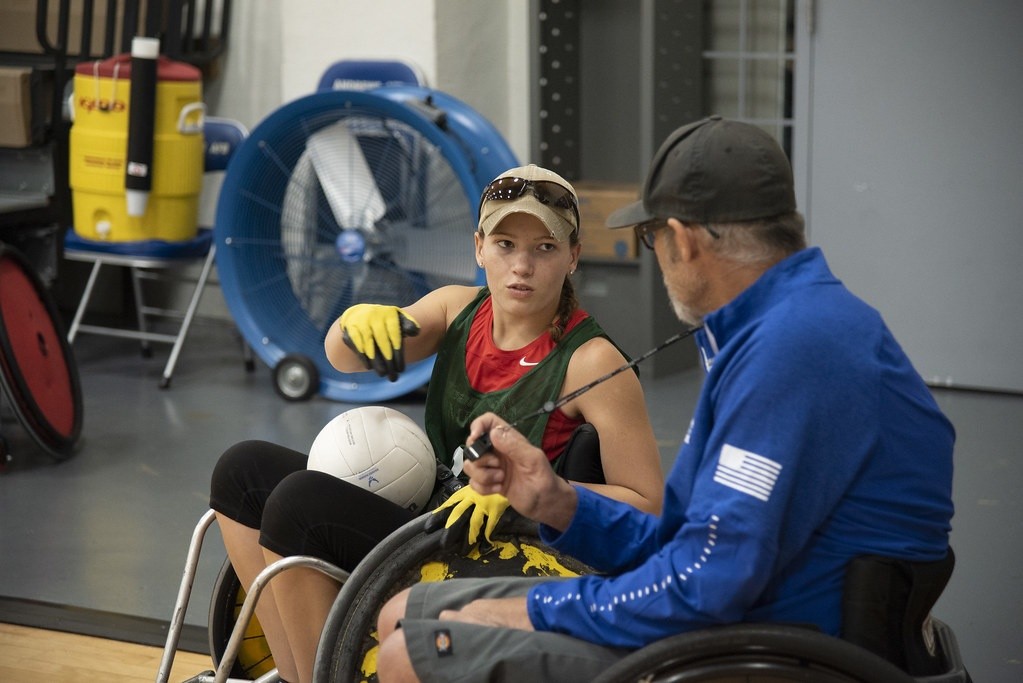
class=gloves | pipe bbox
[339,302,421,382]
[422,484,520,555]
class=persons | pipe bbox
[209,163,663,683]
[376,115,955,683]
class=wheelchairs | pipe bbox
[592,549,970,683]
[155,423,601,683]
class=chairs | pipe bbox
[64,119,252,386]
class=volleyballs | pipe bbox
[306,406,438,516]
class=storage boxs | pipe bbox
[0,68,33,148]
[568,183,640,259]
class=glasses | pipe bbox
[633,220,720,250]
[478,176,580,238]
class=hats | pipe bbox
[477,163,582,243]
[604,115,797,229]
